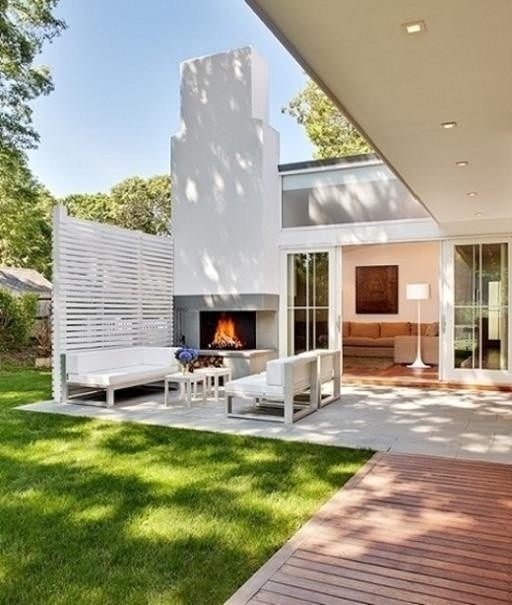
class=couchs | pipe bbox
[224,345,342,425]
[342,335,439,364]
[63,344,178,409]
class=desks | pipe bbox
[193,366,231,402]
[164,371,209,410]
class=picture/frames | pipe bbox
[355,265,398,314]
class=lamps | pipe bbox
[405,282,431,368]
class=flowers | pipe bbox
[174,348,198,367]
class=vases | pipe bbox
[179,363,189,376]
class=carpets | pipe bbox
[343,355,396,370]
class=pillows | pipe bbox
[343,321,439,338]
